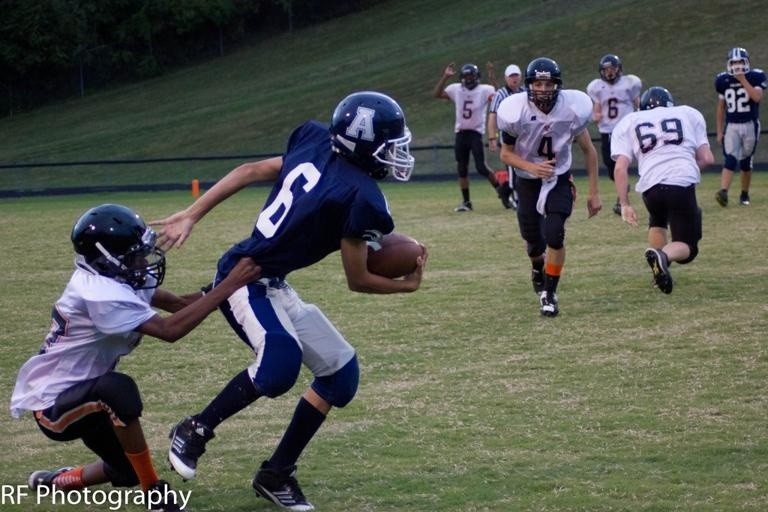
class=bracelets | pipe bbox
[488,136,496,141]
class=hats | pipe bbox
[504,64,522,77]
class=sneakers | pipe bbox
[740,194,749,205]
[715,191,727,206]
[145,480,181,512]
[252,460,314,511]
[645,248,673,294]
[540,290,558,316]
[28,466,76,491]
[453,203,473,211]
[532,270,544,295]
[168,416,215,480]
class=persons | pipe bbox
[486,64,524,209]
[148,92,428,511]
[610,87,714,295]
[434,61,517,212]
[714,48,768,207]
[586,54,642,214]
[11,203,261,512]
[496,57,602,316]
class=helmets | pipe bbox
[640,87,674,110]
[726,48,750,76]
[71,203,166,289]
[459,64,480,90]
[524,57,563,103]
[329,91,415,182]
[598,54,622,84]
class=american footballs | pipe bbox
[367,234,423,278]
